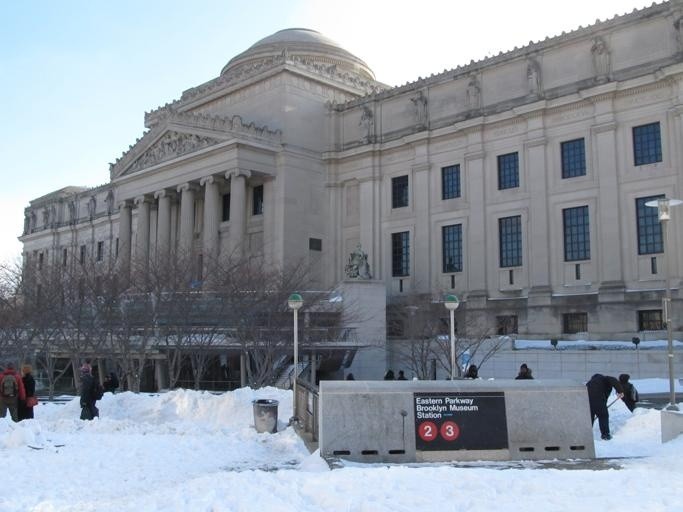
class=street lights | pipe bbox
[286,294,304,421]
[643,197,683,410]
[443,294,460,380]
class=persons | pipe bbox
[616,372,639,412]
[462,364,479,378]
[17,365,36,419]
[514,362,534,378]
[382,369,395,380]
[78,363,100,421]
[346,373,354,380]
[0,362,25,422]
[585,373,624,440]
[397,369,407,380]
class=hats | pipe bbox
[79,365,91,373]
[6,361,15,368]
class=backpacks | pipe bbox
[0,374,19,398]
[85,373,103,400]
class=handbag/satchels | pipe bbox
[26,396,38,407]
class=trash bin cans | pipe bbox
[251,399,280,434]
